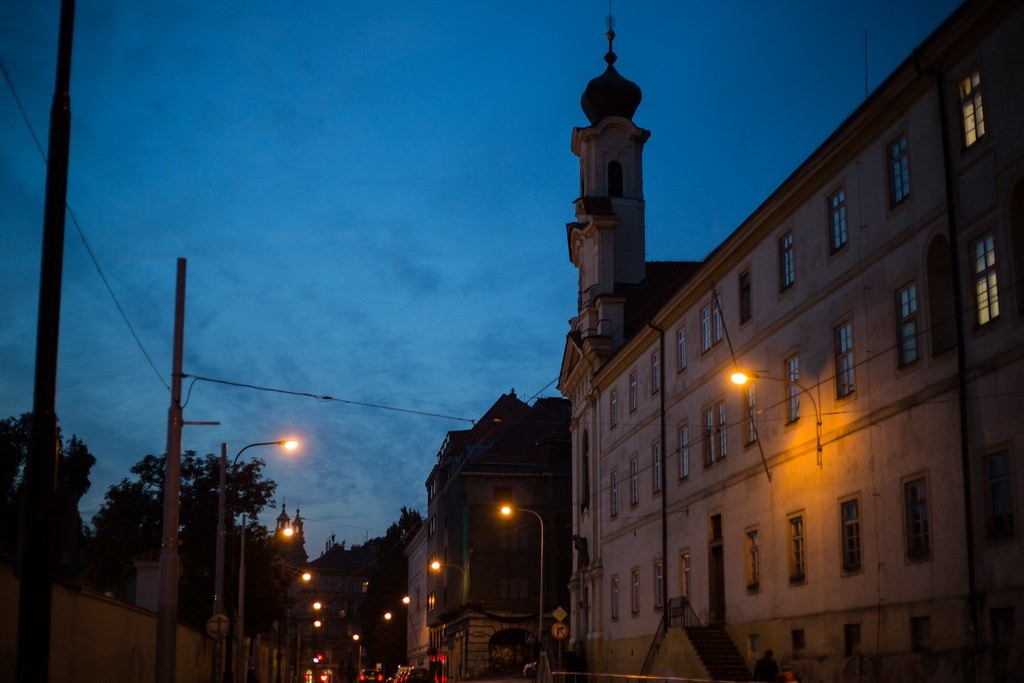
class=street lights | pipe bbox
[502,507,544,683]
[246,526,293,683]
[277,572,321,683]
[223,436,300,683]
[430,562,465,683]
[353,634,361,673]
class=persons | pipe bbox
[753,649,779,683]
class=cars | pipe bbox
[523,661,538,679]
[358,669,379,683]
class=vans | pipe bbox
[392,666,430,683]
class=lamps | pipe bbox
[731,373,822,465]
